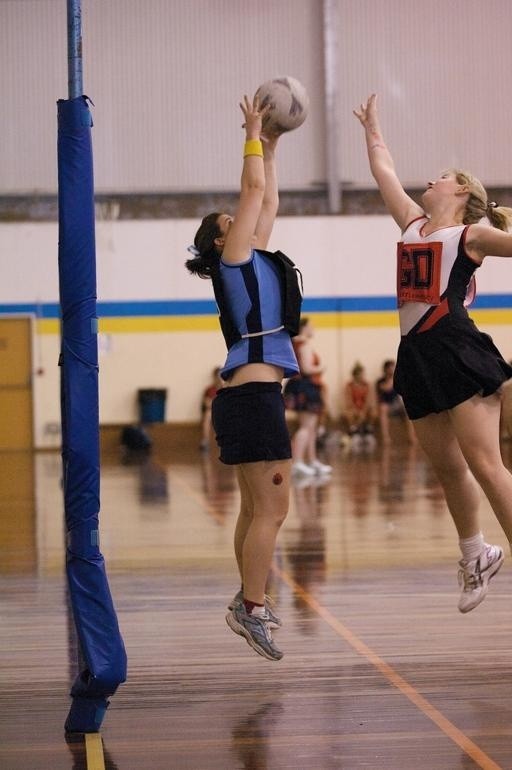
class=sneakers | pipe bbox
[227,589,284,631]
[224,600,285,662]
[459,543,504,614]
[290,460,334,478]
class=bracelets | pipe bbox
[241,138,266,159]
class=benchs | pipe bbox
[98,418,408,458]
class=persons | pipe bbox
[277,315,336,482]
[199,449,237,527]
[284,483,329,637]
[345,448,446,520]
[340,359,378,448]
[198,365,226,451]
[185,91,305,662]
[375,359,422,448]
[352,92,512,617]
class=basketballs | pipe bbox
[253,76,311,136]
